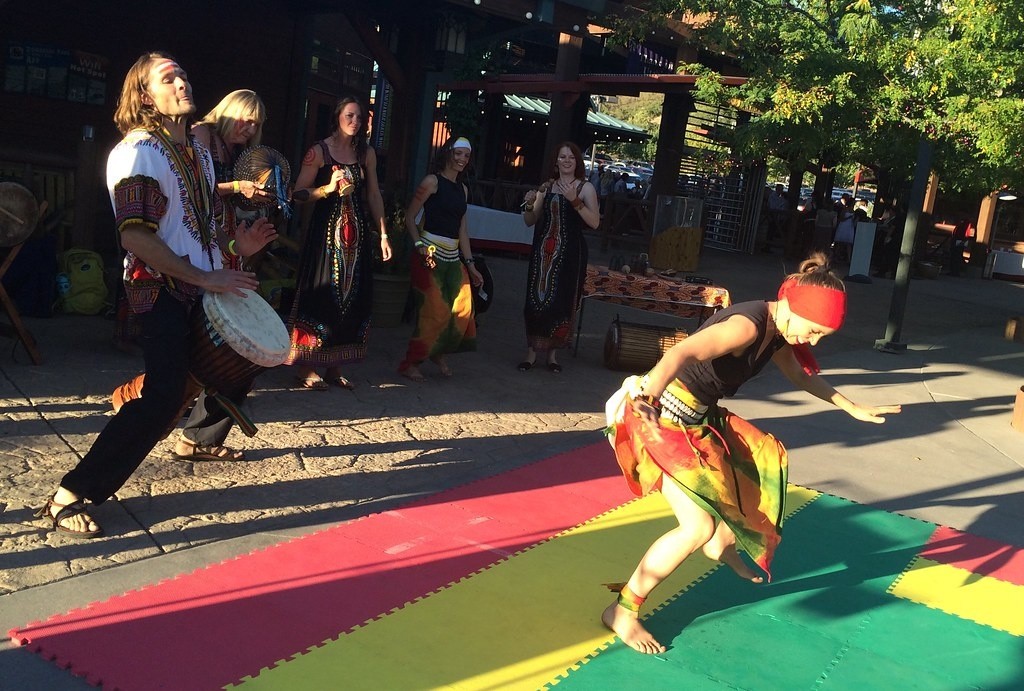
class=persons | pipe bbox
[400,132,485,382]
[601,251,903,656]
[278,95,394,390]
[32,51,293,539]
[516,141,601,373]
[592,165,723,233]
[764,182,869,265]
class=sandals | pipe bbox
[39,490,103,537]
[170,437,245,461]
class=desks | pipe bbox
[991,249,1024,282]
[458,204,535,261]
[569,263,732,359]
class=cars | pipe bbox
[583,155,724,195]
[771,180,876,211]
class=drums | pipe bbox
[0,174,41,248]
[112,286,291,441]
[603,319,690,373]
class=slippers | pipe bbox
[324,372,354,388]
[295,375,328,389]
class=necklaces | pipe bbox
[555,177,578,185]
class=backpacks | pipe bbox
[59,246,108,314]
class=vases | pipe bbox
[372,250,403,274]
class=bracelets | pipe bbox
[524,204,534,213]
[464,257,476,265]
[570,197,582,207]
[414,240,425,248]
[574,202,585,211]
[233,181,240,194]
[228,239,239,256]
[380,233,391,239]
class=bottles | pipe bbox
[57,274,69,294]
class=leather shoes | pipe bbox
[518,359,536,371]
[547,358,560,373]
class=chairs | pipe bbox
[0,186,48,365]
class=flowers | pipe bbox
[368,209,427,264]
